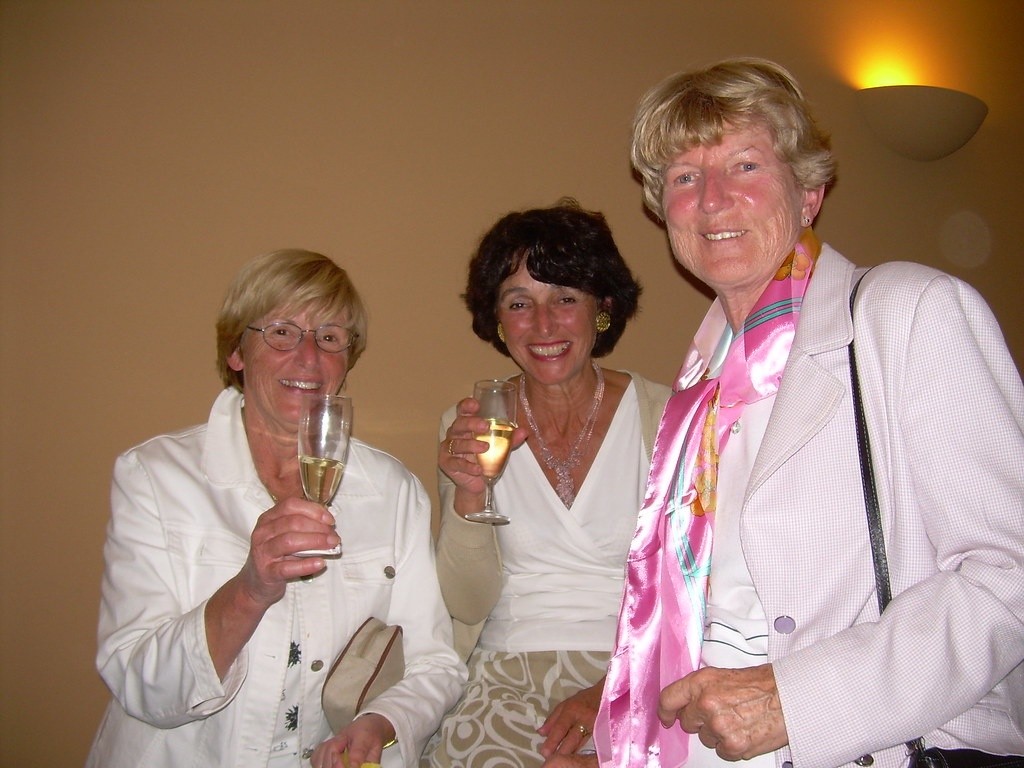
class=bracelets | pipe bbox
[381,737,399,752]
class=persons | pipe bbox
[410,191,679,766]
[587,50,1022,768]
[80,247,470,768]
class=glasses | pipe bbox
[246,322,360,353]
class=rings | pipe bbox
[575,723,589,736]
[447,437,457,456]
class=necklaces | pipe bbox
[511,357,608,510]
[239,410,316,509]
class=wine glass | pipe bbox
[463,380,518,525]
[291,394,352,558]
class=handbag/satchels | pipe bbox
[913,747,1024,768]
[322,617,405,736]
